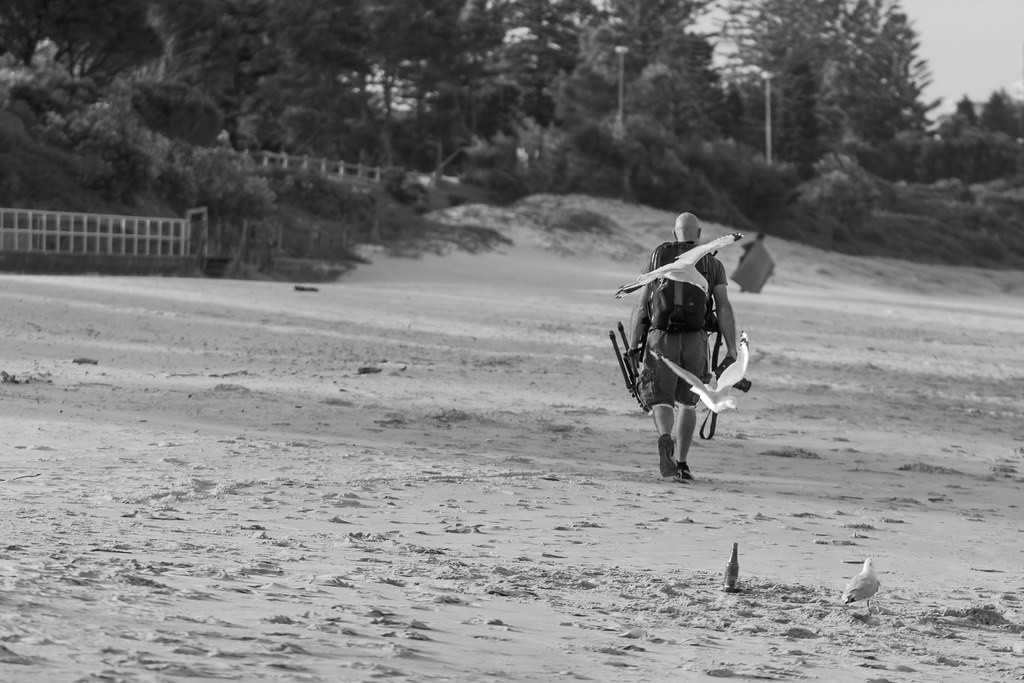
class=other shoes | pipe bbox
[676,468,694,485]
[657,435,679,479]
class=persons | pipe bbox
[628,212,738,481]
[739,233,774,293]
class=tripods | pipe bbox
[608,321,643,408]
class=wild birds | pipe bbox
[841,556,882,606]
[649,330,751,414]
[613,230,746,301]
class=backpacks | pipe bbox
[647,240,713,332]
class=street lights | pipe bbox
[614,46,630,121]
[761,71,773,167]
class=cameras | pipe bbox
[715,356,752,393]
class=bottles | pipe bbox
[723,543,740,592]
